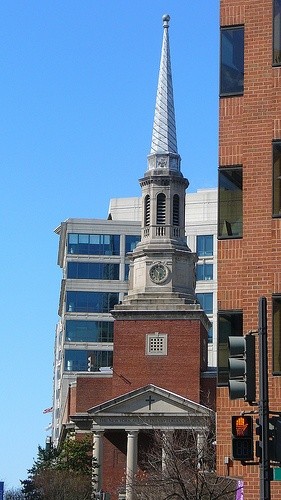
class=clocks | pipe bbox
[149,263,169,284]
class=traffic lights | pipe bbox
[230,415,253,461]
[227,334,256,401]
